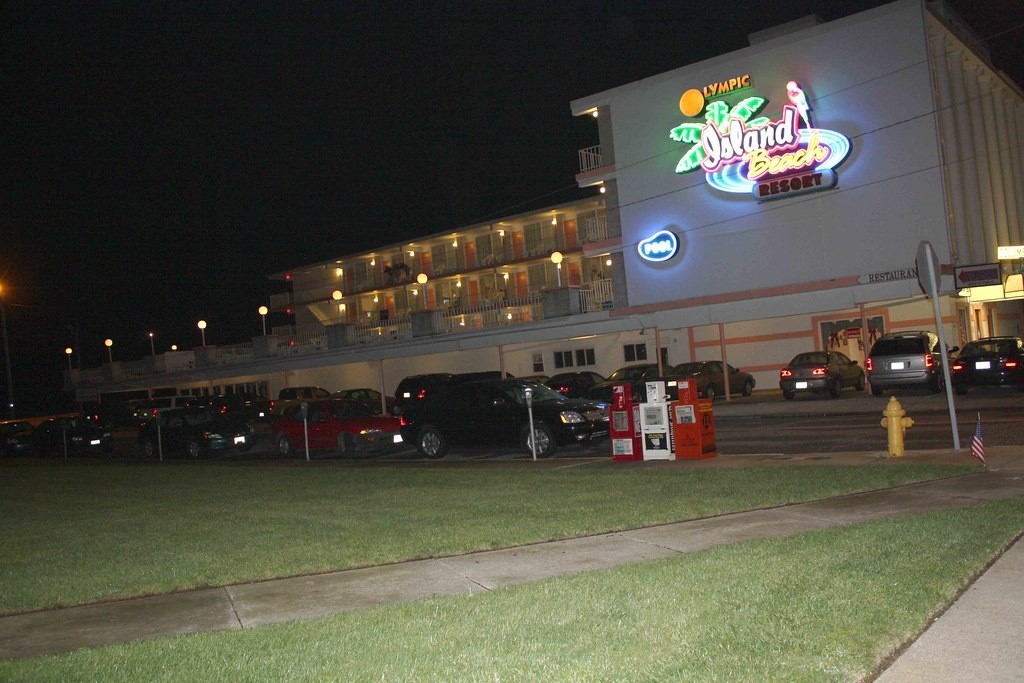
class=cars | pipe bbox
[400,378,611,458]
[27,416,114,458]
[137,406,257,459]
[586,362,674,404]
[275,399,403,457]
[329,387,395,411]
[0,419,37,457]
[950,335,1024,394]
[664,360,755,401]
[544,369,607,399]
[779,350,865,400]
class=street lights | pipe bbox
[149,333,154,355]
[258,306,268,335]
[0,282,17,419]
[104,338,113,363]
[198,319,207,346]
[332,290,342,324]
[65,347,72,377]
[416,272,429,309]
[172,344,177,360]
[551,251,563,287]
[336,267,344,291]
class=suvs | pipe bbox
[394,372,453,409]
[131,395,188,421]
[454,370,515,378]
[279,385,332,400]
[195,393,265,423]
[83,402,139,431]
[866,330,959,395]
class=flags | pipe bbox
[969,421,986,465]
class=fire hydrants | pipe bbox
[879,394,915,457]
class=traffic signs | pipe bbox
[953,262,1002,289]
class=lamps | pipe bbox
[416,273,427,309]
[65,303,268,370]
[331,290,342,322]
[550,251,563,286]
